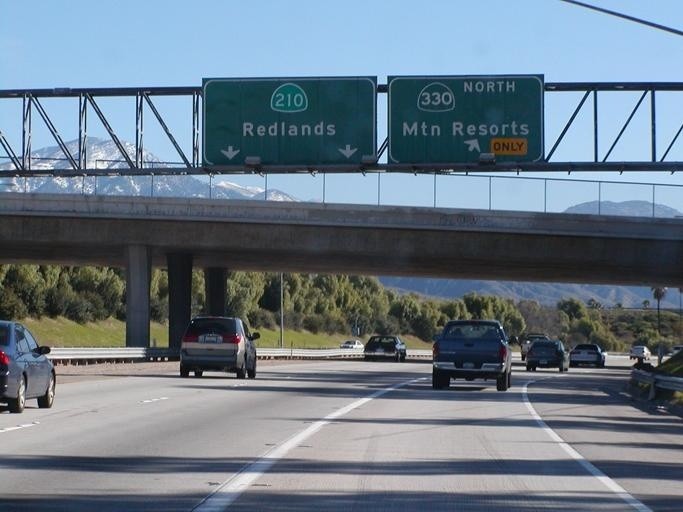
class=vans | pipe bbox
[180,316,260,380]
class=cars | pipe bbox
[520,333,606,373]
[340,339,364,350]
[629,345,650,360]
[363,334,406,360]
[0,321,55,414]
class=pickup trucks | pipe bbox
[430,320,512,392]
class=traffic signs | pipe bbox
[203,74,377,170]
[387,75,546,165]
